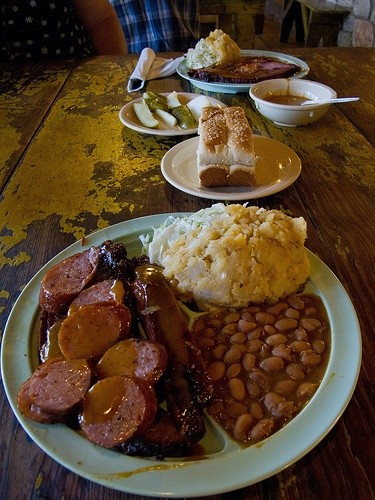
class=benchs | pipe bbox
[280,0,351,48]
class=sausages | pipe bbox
[17,247,213,453]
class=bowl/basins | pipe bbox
[249,78,338,127]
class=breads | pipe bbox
[196,105,257,187]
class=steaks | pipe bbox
[185,55,298,83]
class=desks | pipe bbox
[0,47,375,500]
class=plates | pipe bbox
[176,50,310,94]
[118,92,227,136]
[0,212,363,498]
[160,134,302,200]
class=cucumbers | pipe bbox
[132,91,196,129]
